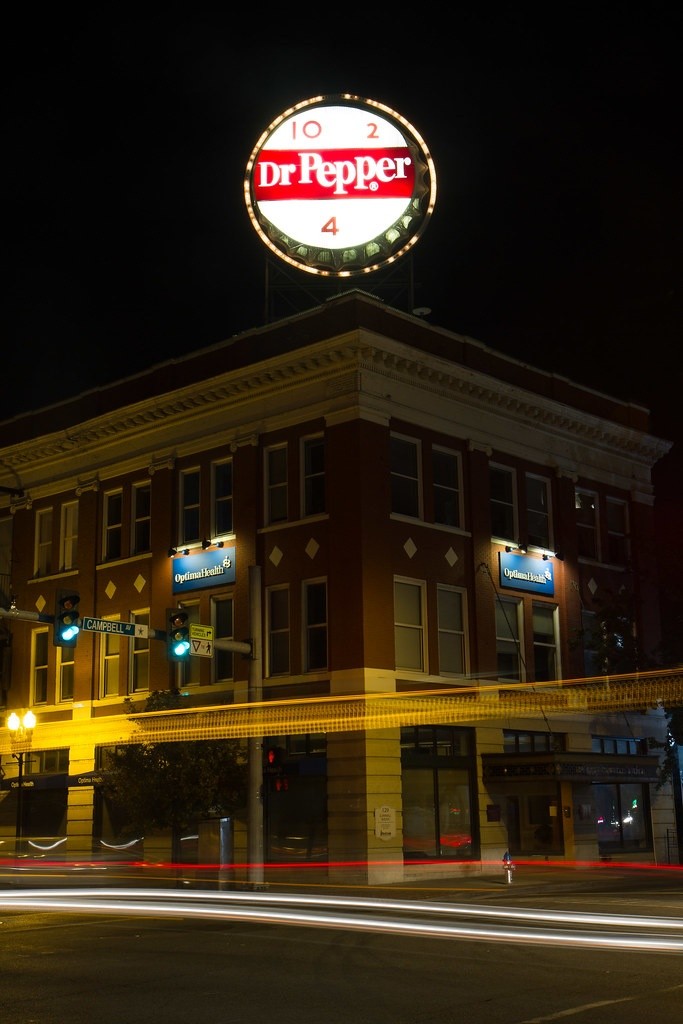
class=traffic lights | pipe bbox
[170,612,190,657]
[58,594,80,643]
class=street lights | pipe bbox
[6,710,36,852]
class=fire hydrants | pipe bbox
[501,851,516,883]
